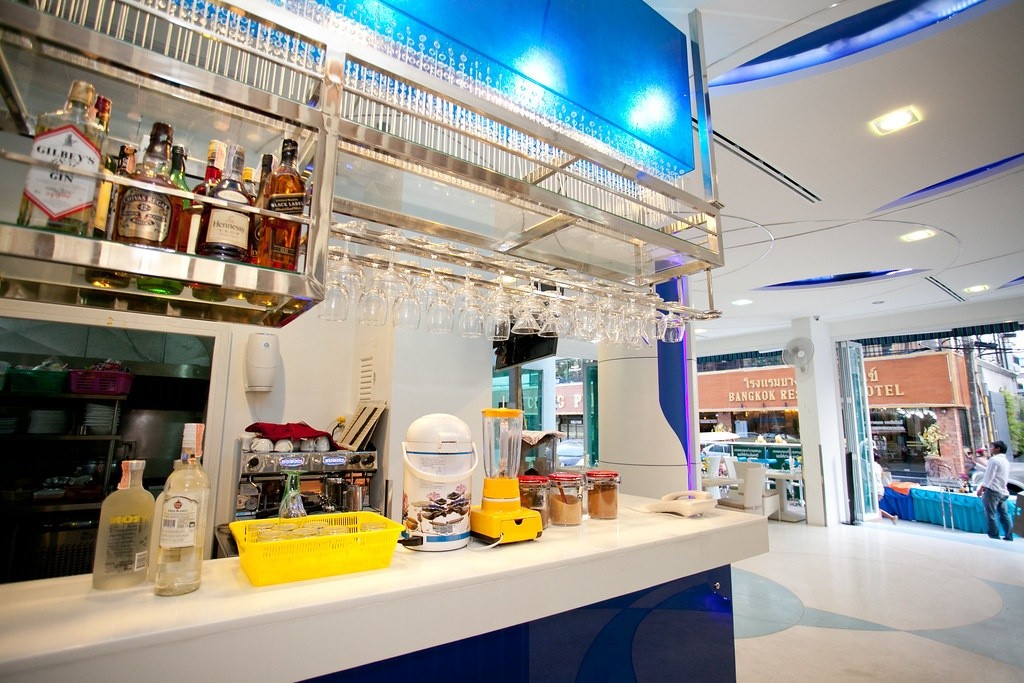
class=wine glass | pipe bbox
[318,219,684,350]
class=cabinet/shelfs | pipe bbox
[0,389,126,512]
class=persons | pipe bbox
[967,440,1014,541]
[873,453,898,525]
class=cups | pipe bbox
[346,486,363,513]
[241,434,330,453]
[248,522,387,543]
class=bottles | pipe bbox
[93,459,155,591]
[279,470,307,517]
[16,79,309,324]
[147,423,211,597]
[964,480,973,493]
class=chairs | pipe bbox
[705,455,780,525]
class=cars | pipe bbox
[701,442,731,455]
[545,439,585,466]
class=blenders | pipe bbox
[470,406,543,545]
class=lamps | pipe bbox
[941,408,947,414]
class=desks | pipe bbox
[882,485,1022,533]
[766,469,806,523]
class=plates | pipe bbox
[0,410,65,433]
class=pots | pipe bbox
[115,408,198,478]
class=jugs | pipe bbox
[401,413,478,551]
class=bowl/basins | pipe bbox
[82,403,121,436]
[43,475,71,487]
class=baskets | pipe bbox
[228,510,406,587]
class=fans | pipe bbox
[781,337,816,373]
